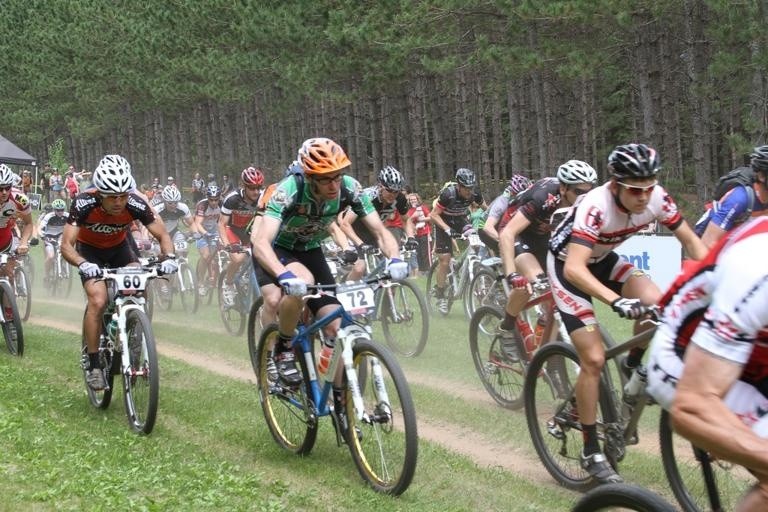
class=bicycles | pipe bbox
[523,303,758,512]
[81,260,178,437]
[256,272,419,498]
[566,480,681,512]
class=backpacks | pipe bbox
[711,166,757,225]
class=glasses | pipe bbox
[566,186,593,196]
[616,180,658,194]
[309,174,344,184]
[98,194,130,199]
[245,185,262,190]
[0,187,11,191]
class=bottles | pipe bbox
[318,334,334,374]
[237,274,248,287]
[623,364,647,402]
[532,314,546,346]
[110,313,118,342]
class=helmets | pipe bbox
[607,143,661,180]
[0,163,13,186]
[557,159,598,185]
[750,144,768,168]
[52,199,66,209]
[94,153,138,194]
[240,165,264,185]
[297,136,351,174]
[510,174,532,193]
[161,186,182,202]
[377,166,404,191]
[456,168,476,188]
[206,185,221,198]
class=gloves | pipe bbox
[276,270,307,297]
[386,258,410,280]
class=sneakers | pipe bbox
[222,279,236,307]
[579,450,624,484]
[81,357,107,390]
[436,298,449,314]
[265,344,302,386]
[156,275,217,302]
[495,318,522,363]
